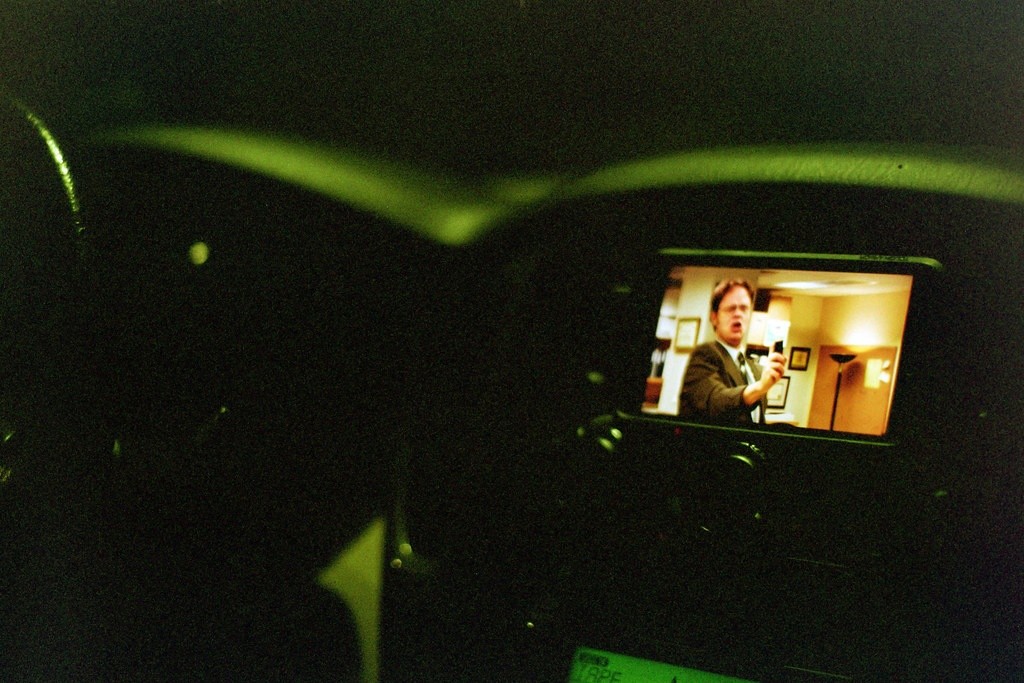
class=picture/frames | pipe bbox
[789,344,813,371]
[766,376,791,409]
[674,316,702,354]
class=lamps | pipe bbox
[826,354,858,431]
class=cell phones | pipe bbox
[612,246,944,448]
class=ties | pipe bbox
[738,353,748,385]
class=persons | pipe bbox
[676,276,787,427]
